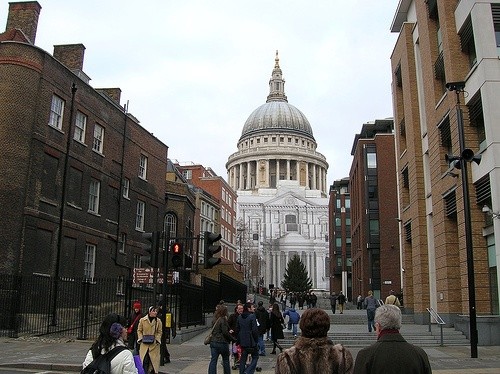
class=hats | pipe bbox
[133,302,141,309]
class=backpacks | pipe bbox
[80,344,129,374]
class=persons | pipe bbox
[127,303,147,355]
[228,304,244,368]
[385,289,400,306]
[208,306,238,374]
[216,300,225,311]
[283,309,300,336]
[364,291,380,332]
[269,304,284,354]
[354,304,432,374]
[356,294,363,309]
[253,301,269,356]
[337,292,346,314]
[275,309,353,374]
[83,313,138,374]
[155,305,176,366]
[329,293,337,314]
[235,302,259,374]
[269,293,317,310]
[137,306,162,374]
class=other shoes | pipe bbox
[159,357,164,366]
[164,356,170,363]
[270,350,276,355]
[279,347,283,353]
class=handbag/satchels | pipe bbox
[280,322,286,329]
[204,334,212,345]
[127,325,133,333]
[142,334,155,344]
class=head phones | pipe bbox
[110,314,122,339]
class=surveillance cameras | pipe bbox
[482,206,490,213]
